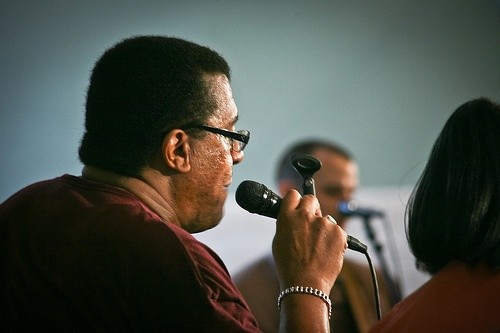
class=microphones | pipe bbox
[236,180,367,254]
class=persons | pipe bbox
[234,139,402,333]
[365,97,500,333]
[0,35,348,333]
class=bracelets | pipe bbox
[277,286,332,320]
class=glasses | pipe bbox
[180,115,251,152]
